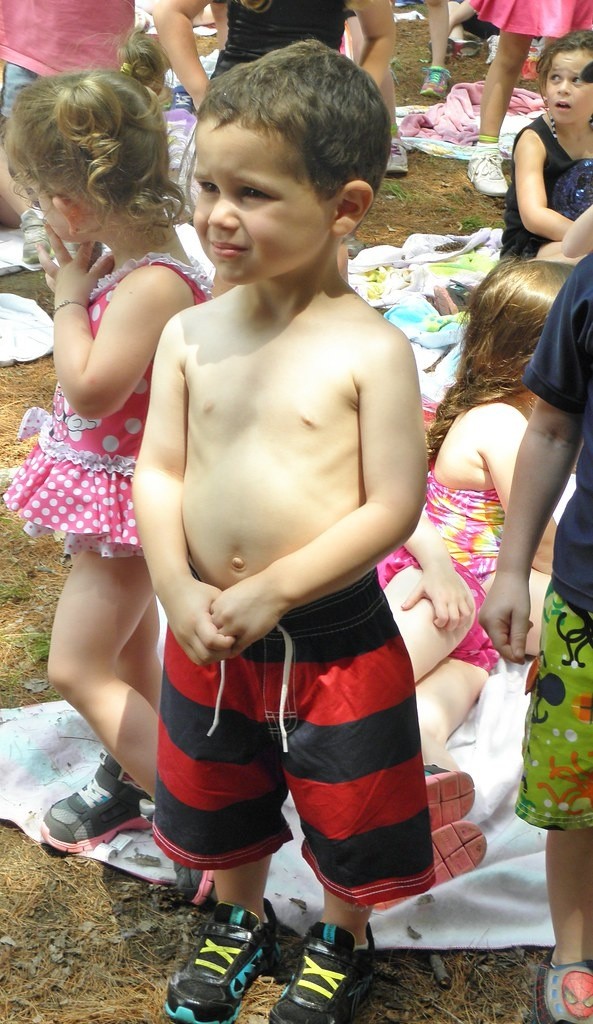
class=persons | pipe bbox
[427,260,574,662]
[467,0,592,198]
[149,0,396,111]
[341,11,408,172]
[428,0,502,63]
[560,203,593,260]
[1,1,230,264]
[475,251,593,1024]
[131,37,429,1024]
[419,0,452,98]
[501,29,593,259]
[0,67,215,907]
[375,514,499,768]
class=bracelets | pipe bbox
[50,300,90,318]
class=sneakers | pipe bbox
[268,921,376,1024]
[386,138,408,174]
[467,142,509,197]
[173,860,214,905]
[41,748,153,853]
[19,211,55,263]
[420,67,451,99]
[486,35,501,64]
[163,898,280,1024]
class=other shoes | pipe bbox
[429,37,480,57]
[425,764,476,831]
[365,820,487,912]
[534,944,593,1024]
